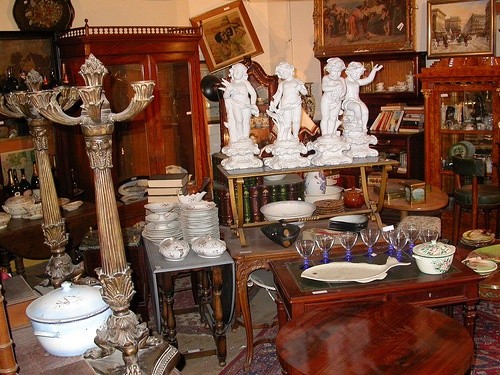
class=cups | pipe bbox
[22,203,42,216]
[0,212,13,229]
[376,82,408,91]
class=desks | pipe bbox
[218,156,500,375]
[0,198,235,375]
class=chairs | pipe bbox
[452,155,500,246]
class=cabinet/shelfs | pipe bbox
[316,51,426,180]
[55,18,214,229]
[218,60,278,151]
[415,56,500,241]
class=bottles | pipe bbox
[305,170,326,195]
[0,154,79,212]
[344,187,365,208]
[0,64,58,138]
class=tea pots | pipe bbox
[404,71,413,91]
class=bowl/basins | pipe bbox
[261,224,301,246]
[260,200,316,219]
[412,240,455,275]
[25,282,113,357]
[2,192,39,218]
[158,235,226,261]
[305,185,344,204]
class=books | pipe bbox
[370,104,427,173]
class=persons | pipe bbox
[219,58,383,145]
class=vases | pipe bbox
[301,82,315,120]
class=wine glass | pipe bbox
[294,225,438,271]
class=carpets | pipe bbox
[218,302,500,375]
[131,276,262,337]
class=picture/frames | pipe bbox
[0,30,61,184]
[197,60,221,124]
[426,0,496,59]
[189,0,265,74]
[312,0,416,58]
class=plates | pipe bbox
[314,198,345,217]
[12,0,75,35]
[299,256,412,283]
[142,201,221,247]
[460,230,500,274]
[28,198,83,218]
[328,214,370,235]
